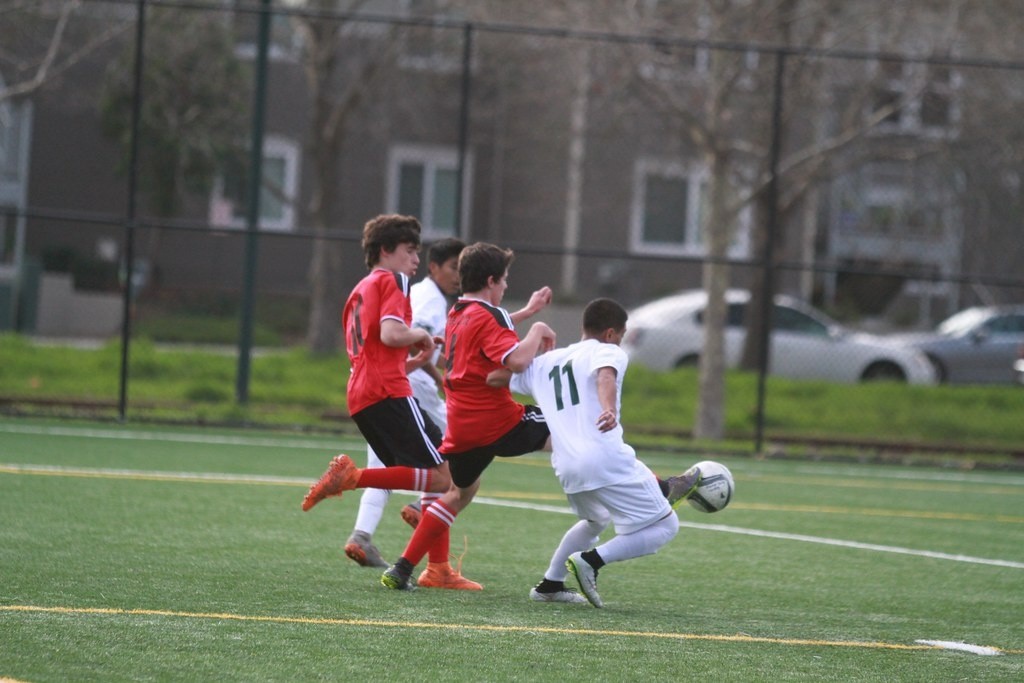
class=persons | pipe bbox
[382,244,701,589]
[485,298,680,608]
[344,237,470,569]
[302,214,484,592]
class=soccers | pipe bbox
[683,459,735,514]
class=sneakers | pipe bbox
[301,454,363,512]
[344,530,391,568]
[565,551,603,608]
[400,498,422,529]
[530,588,586,602]
[417,535,483,590]
[667,466,702,511]
[381,565,422,592]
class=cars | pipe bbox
[621,289,937,387]
[895,307,1024,385]
